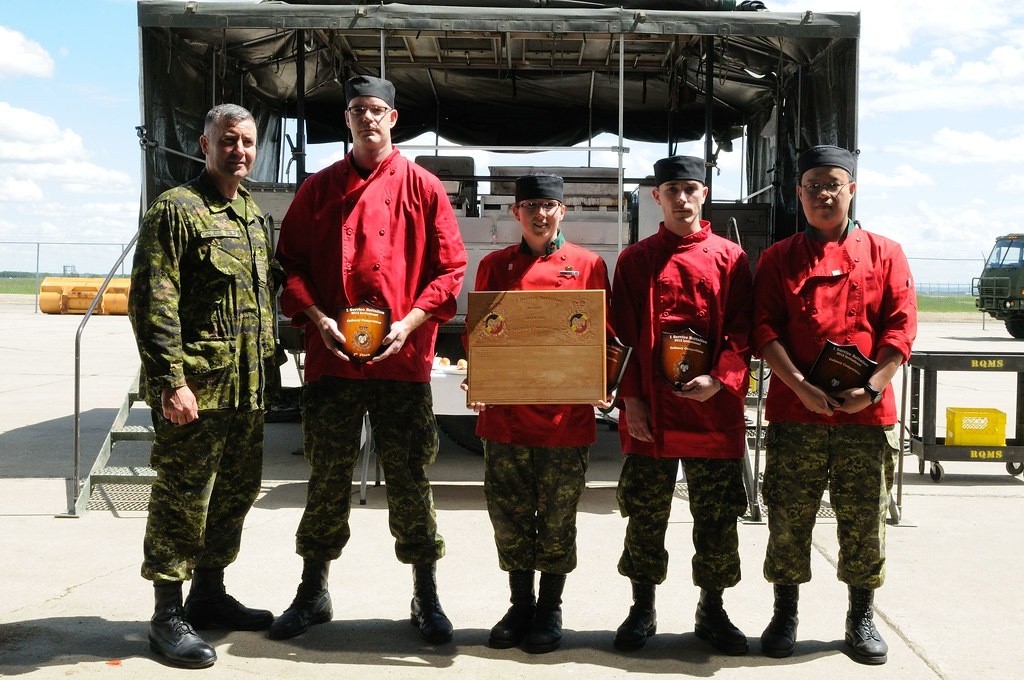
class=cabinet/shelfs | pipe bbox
[705,203,772,283]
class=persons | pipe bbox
[612,156,750,649]
[750,144,916,664]
[272,76,466,644]
[132,103,272,669]
[458,175,621,653]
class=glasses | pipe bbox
[347,106,391,116]
[518,202,562,210]
[802,182,850,195]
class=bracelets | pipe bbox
[720,381,726,391]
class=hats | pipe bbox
[654,156,706,187]
[514,172,564,203]
[345,75,395,108]
[798,144,854,177]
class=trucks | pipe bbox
[37,0,902,522]
[971,235,1024,339]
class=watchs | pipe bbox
[864,381,882,404]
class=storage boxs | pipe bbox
[945,408,1007,446]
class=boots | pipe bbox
[147,580,217,670]
[268,557,332,639]
[183,566,274,633]
[489,569,567,654]
[844,589,888,663]
[760,583,800,656]
[614,579,656,653]
[409,562,453,645]
[693,587,749,653]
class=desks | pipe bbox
[360,364,620,505]
